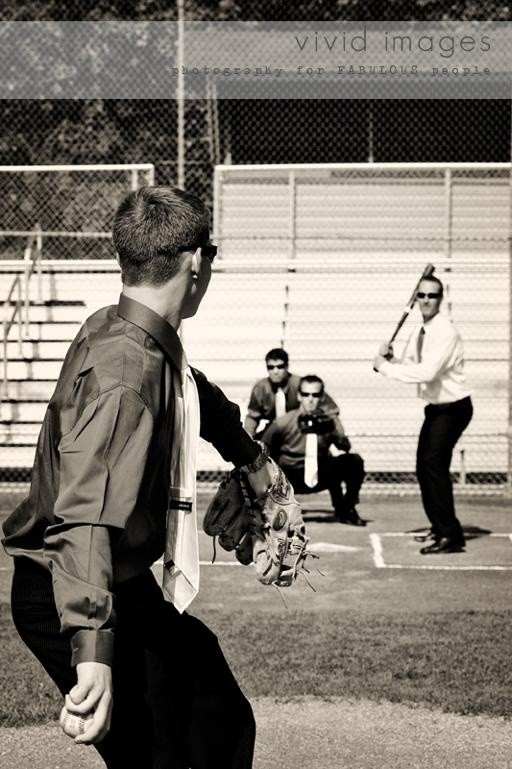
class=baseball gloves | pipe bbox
[202,440,308,587]
[298,410,337,435]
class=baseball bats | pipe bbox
[372,263,436,372]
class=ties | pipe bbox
[304,433,319,488]
[164,353,200,615]
[275,388,286,416]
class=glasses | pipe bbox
[268,364,285,371]
[418,292,437,299]
[178,244,217,262]
[301,392,319,397]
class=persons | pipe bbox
[373,275,473,554]
[244,348,367,506]
[2,184,310,769]
[260,375,368,526]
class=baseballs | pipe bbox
[59,706,94,739]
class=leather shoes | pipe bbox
[414,532,437,542]
[421,538,465,554]
[344,510,365,525]
